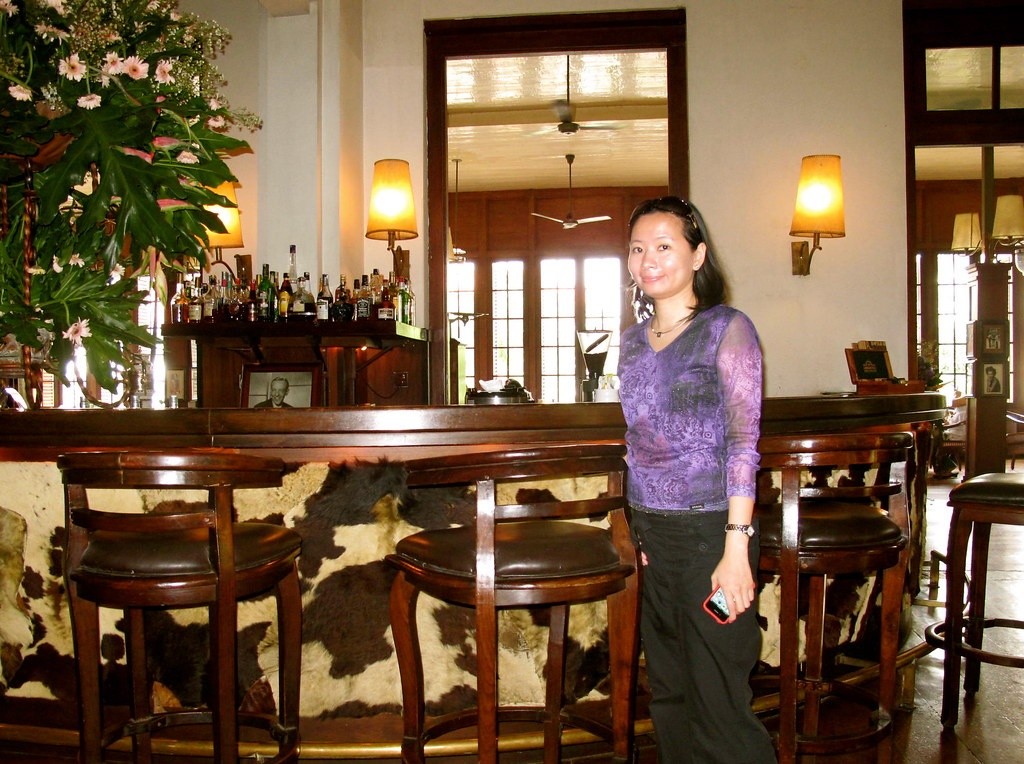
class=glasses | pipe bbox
[628,196,702,239]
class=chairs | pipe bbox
[939,420,966,470]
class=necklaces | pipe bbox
[651,312,692,338]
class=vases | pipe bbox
[0,316,56,409]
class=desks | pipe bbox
[161,318,428,410]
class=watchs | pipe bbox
[725,524,755,537]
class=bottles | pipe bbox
[170,245,316,322]
[317,269,415,327]
[131,353,153,395]
[582,380,599,401]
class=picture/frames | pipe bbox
[165,369,187,402]
[240,364,322,407]
[964,320,1010,398]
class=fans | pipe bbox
[531,154,613,230]
[529,56,622,135]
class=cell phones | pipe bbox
[703,582,755,624]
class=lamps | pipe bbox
[988,195,1024,281]
[789,154,845,276]
[365,159,419,281]
[951,212,982,263]
[202,179,251,285]
[577,330,613,401]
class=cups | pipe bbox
[577,329,613,380]
[594,389,620,402]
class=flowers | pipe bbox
[0,0,263,410]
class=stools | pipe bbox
[753,431,913,764]
[57,451,301,764]
[385,442,644,763]
[925,473,1024,727]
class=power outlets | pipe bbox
[393,371,409,387]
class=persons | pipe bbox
[984,366,1000,392]
[617,196,779,764]
[985,328,1000,349]
[253,376,293,408]
